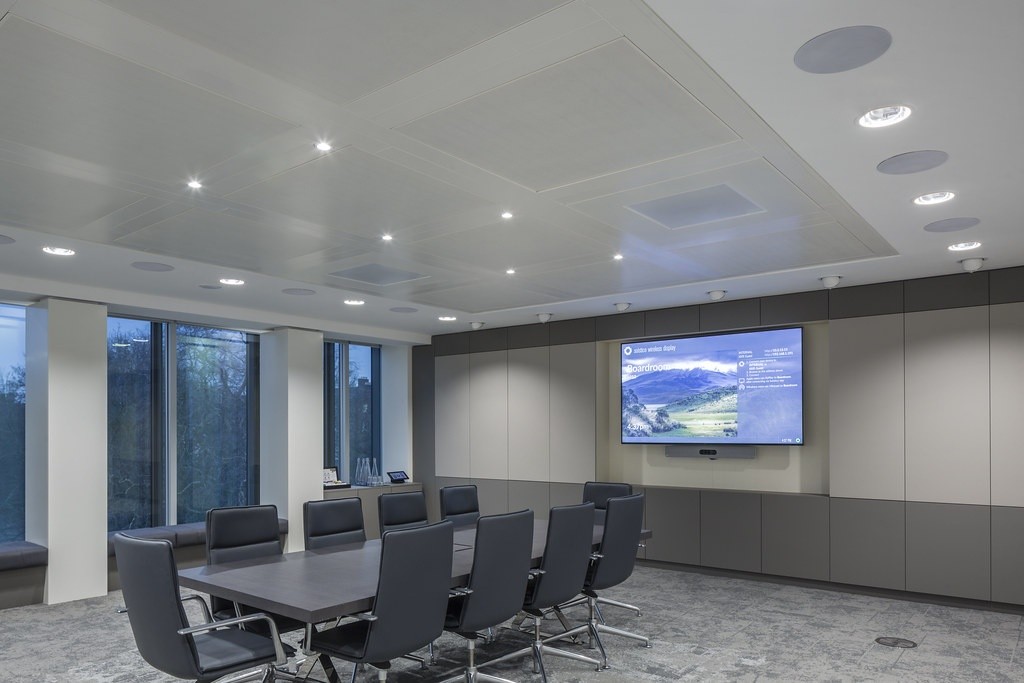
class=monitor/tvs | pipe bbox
[620,326,806,447]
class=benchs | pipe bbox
[0,517,287,613]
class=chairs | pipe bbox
[110,482,645,683]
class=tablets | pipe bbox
[388,471,409,480]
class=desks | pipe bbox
[174,519,655,683]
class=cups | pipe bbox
[367,475,384,487]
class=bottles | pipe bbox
[354,457,379,486]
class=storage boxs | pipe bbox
[323,467,350,489]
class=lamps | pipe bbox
[471,322,482,329]
[961,259,983,274]
[823,277,839,288]
[709,290,725,301]
[538,313,550,324]
[615,303,630,312]
